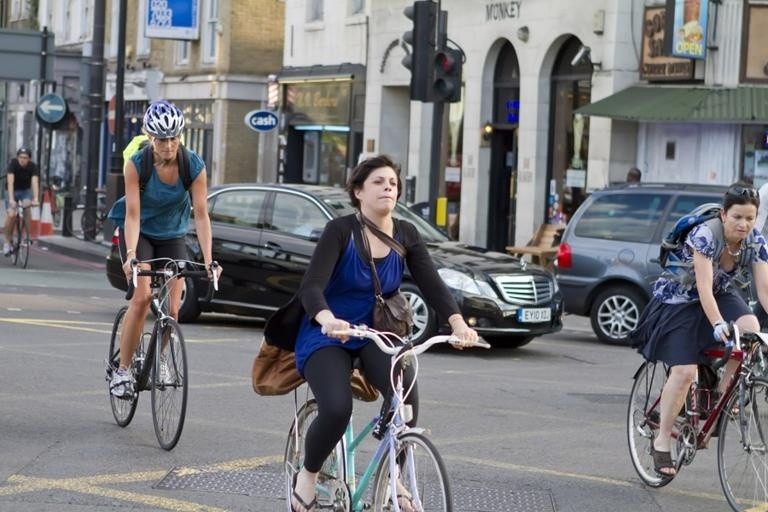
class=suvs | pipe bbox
[553,182,749,347]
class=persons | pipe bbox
[109,99,226,399]
[263,154,477,512]
[1,146,41,257]
[627,183,768,512]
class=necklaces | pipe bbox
[724,238,744,257]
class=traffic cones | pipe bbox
[30,189,55,237]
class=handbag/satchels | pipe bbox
[252,337,379,402]
[373,291,414,340]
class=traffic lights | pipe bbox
[431,48,462,102]
[400,2,415,72]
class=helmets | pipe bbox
[143,99,185,140]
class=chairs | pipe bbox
[505,223,568,266]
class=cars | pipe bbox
[105,184,566,351]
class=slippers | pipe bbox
[290,470,317,512]
[389,494,418,511]
[650,439,675,476]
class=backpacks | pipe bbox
[659,202,755,290]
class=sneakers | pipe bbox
[110,365,136,399]
[158,355,174,385]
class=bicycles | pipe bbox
[627,320,768,512]
[4,199,33,269]
[283,324,492,511]
[104,257,223,450]
[66,193,114,244]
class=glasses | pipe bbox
[728,186,758,198]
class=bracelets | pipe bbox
[452,316,466,325]
[126,250,135,255]
